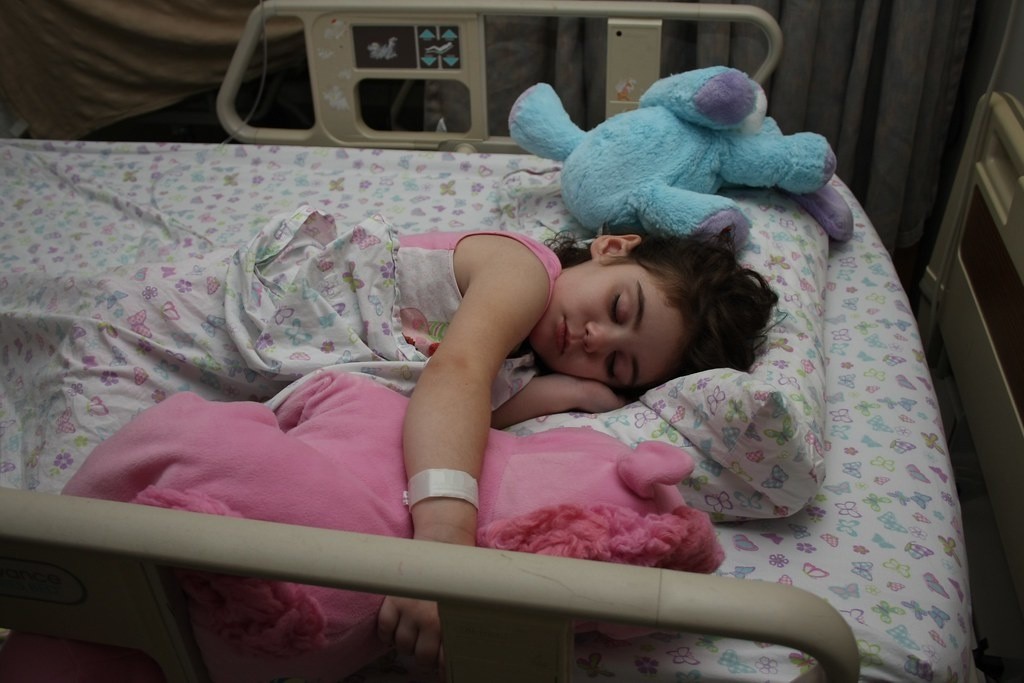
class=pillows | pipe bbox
[498,166,830,524]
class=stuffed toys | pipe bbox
[0,373,725,683]
[508,67,853,248]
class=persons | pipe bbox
[0,205,780,682]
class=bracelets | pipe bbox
[403,468,479,512]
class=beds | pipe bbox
[0,138,989,683]
